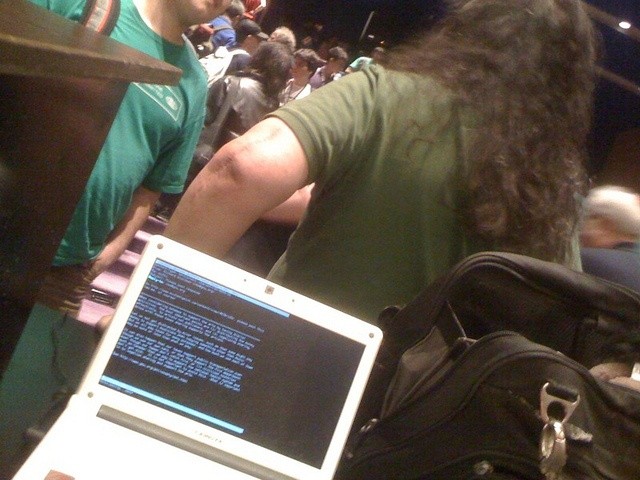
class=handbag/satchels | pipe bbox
[193,75,240,168]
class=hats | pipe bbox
[237,19,269,42]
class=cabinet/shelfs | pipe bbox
[0,0,183,385]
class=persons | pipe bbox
[97,0,609,442]
[182,0,383,186]
[1,0,229,480]
[573,181,638,306]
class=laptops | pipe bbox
[11,232,384,480]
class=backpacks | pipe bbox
[333,252,640,480]
[187,24,234,59]
